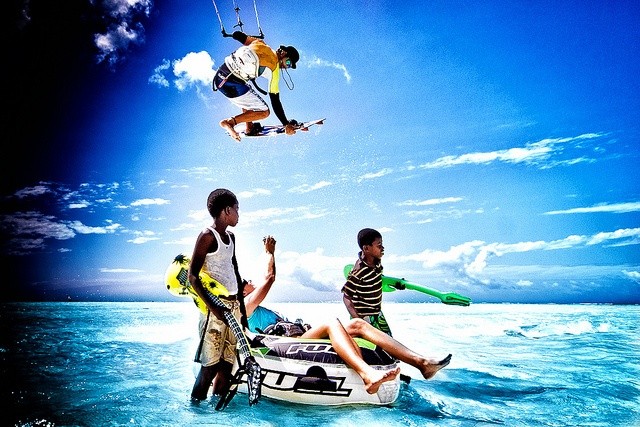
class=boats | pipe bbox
[191,329,401,405]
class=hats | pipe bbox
[280,44,299,69]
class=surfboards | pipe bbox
[224,117,326,138]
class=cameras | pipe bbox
[262,238,276,244]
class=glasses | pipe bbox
[242,278,249,289]
[285,58,292,69]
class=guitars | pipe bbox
[343,264,472,307]
[165,254,262,406]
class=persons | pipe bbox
[240,236,452,393]
[188,189,250,401]
[342,229,404,340]
[213,31,299,141]
